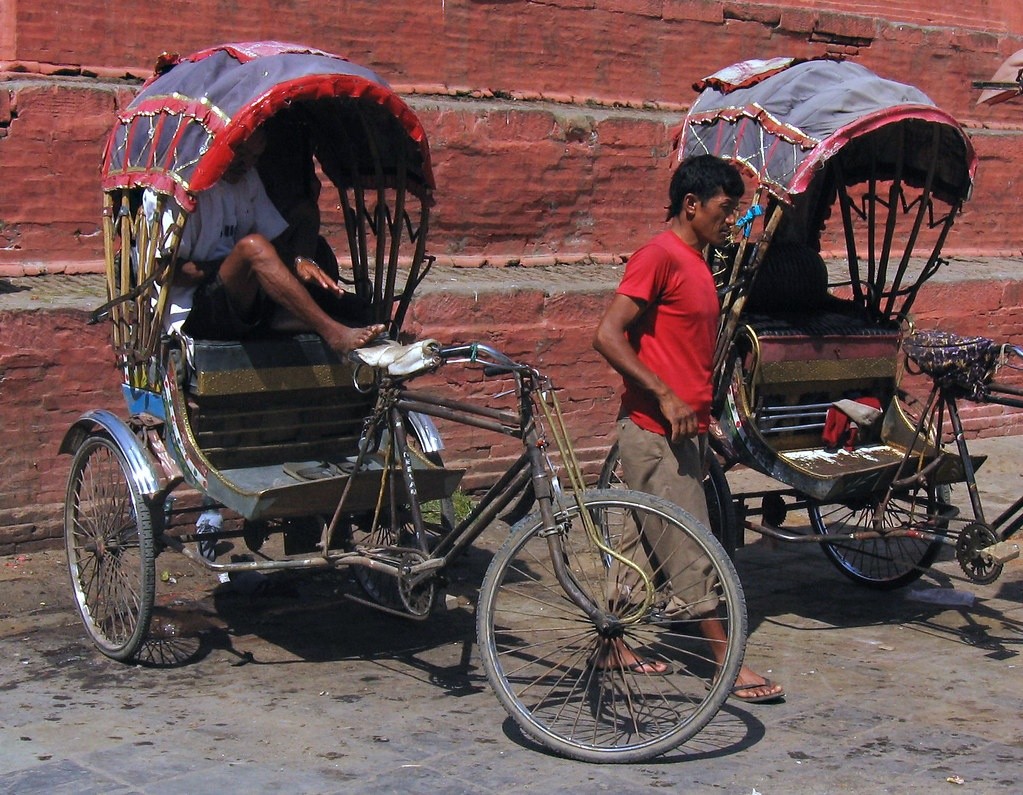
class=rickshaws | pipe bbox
[592,53,1023,617]
[54,38,748,764]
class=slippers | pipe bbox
[585,653,674,675]
[704,676,788,702]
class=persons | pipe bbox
[134,125,386,357]
[586,154,784,705]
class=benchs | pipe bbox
[724,241,900,363]
[129,235,378,372]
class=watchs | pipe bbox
[293,254,320,268]
